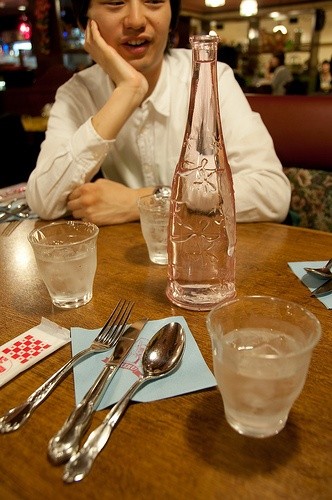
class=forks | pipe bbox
[0,202,29,215]
[0,296,136,434]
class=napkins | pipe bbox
[71,316,217,411]
[288,261,332,309]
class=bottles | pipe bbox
[164,34,238,312]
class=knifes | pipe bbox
[47,317,150,467]
[309,279,332,298]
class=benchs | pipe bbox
[245,93,332,172]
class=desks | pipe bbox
[0,183,332,500]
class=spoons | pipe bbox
[304,258,332,279]
[62,321,187,484]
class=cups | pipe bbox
[205,294,322,439]
[26,219,99,310]
[136,193,171,266]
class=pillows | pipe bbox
[283,168,332,233]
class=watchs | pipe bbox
[155,184,172,215]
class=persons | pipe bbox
[316,60,332,92]
[259,48,293,96]
[26,0,291,226]
